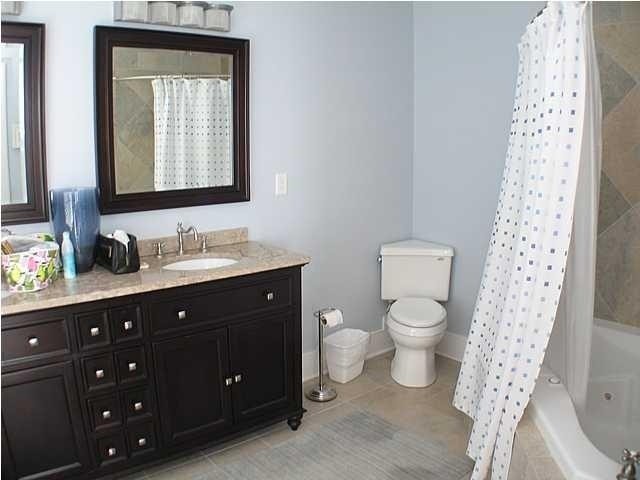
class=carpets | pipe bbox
[189,382,475,480]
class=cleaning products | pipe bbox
[61,232,76,280]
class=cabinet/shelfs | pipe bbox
[147,264,301,336]
[1,361,88,479]
[148,308,302,456]
[69,290,161,478]
[1,302,74,375]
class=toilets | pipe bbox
[379,239,456,389]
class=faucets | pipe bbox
[177,222,199,255]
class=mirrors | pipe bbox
[1,21,49,225]
[94,26,250,216]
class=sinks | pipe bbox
[162,254,238,272]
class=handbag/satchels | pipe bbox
[95,233,140,274]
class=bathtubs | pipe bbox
[531,313,640,480]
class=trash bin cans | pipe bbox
[326,328,371,384]
